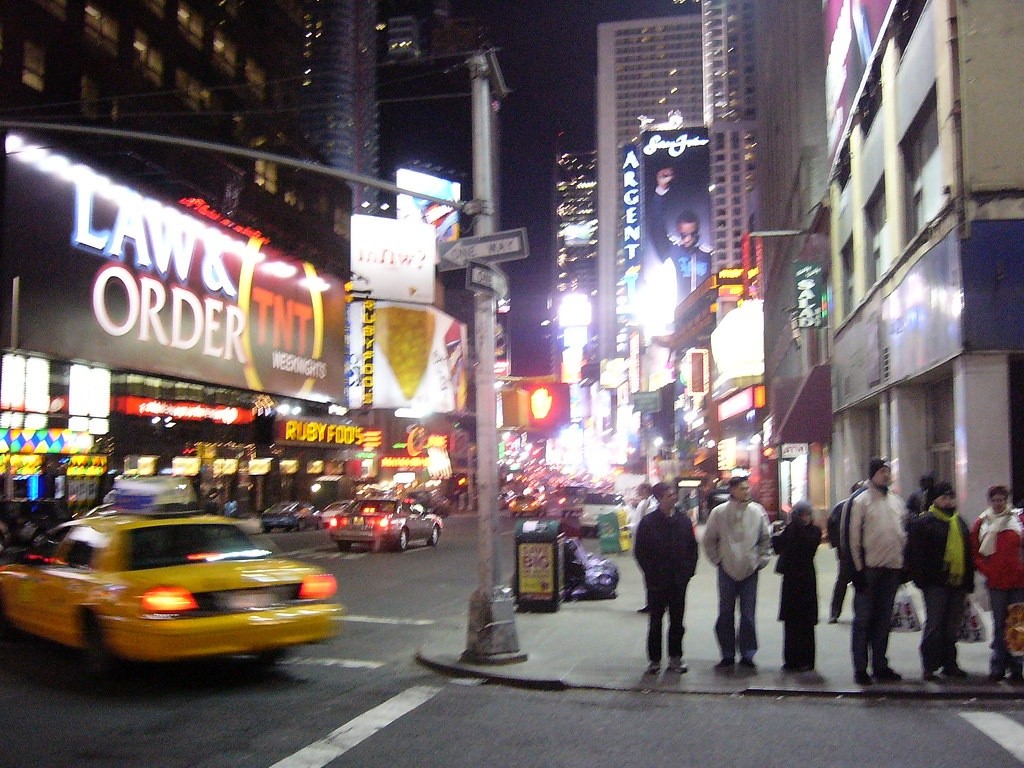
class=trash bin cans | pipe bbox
[515,518,565,612]
[597,511,633,553]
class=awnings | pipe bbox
[772,359,834,446]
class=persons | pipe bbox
[631,483,669,614]
[701,477,774,673]
[634,483,698,675]
[829,456,909,686]
[647,165,712,304]
[905,472,974,681]
[773,496,822,671]
[971,484,1024,689]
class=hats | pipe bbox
[868,459,892,479]
[928,481,954,502]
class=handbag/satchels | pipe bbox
[958,596,987,644]
[889,583,926,631]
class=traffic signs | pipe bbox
[438,228,529,273]
[465,260,508,301]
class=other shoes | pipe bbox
[648,660,660,672]
[738,657,754,671]
[829,617,837,624]
[942,669,967,677]
[670,664,688,674]
[714,657,736,673]
[854,671,872,684]
[990,672,1004,681]
[1010,672,1024,684]
[874,665,902,682]
[923,671,936,681]
[637,604,665,613]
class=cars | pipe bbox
[0,510,346,680]
[558,484,626,537]
[329,496,444,552]
[258,500,321,531]
[314,500,355,530]
[508,494,543,518]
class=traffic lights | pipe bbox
[528,382,570,429]
